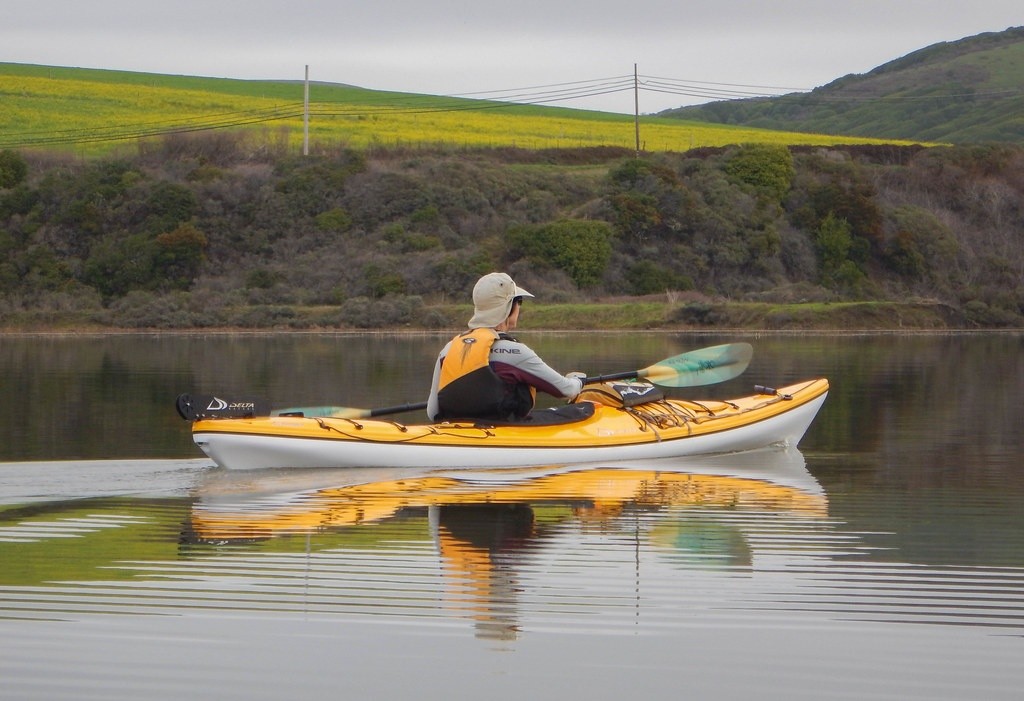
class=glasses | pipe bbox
[514,296,522,307]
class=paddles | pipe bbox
[272,340,755,420]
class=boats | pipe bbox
[176,444,829,542]
[176,377,829,471]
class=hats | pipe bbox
[467,272,536,331]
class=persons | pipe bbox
[426,271,589,423]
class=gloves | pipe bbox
[565,371,587,389]
